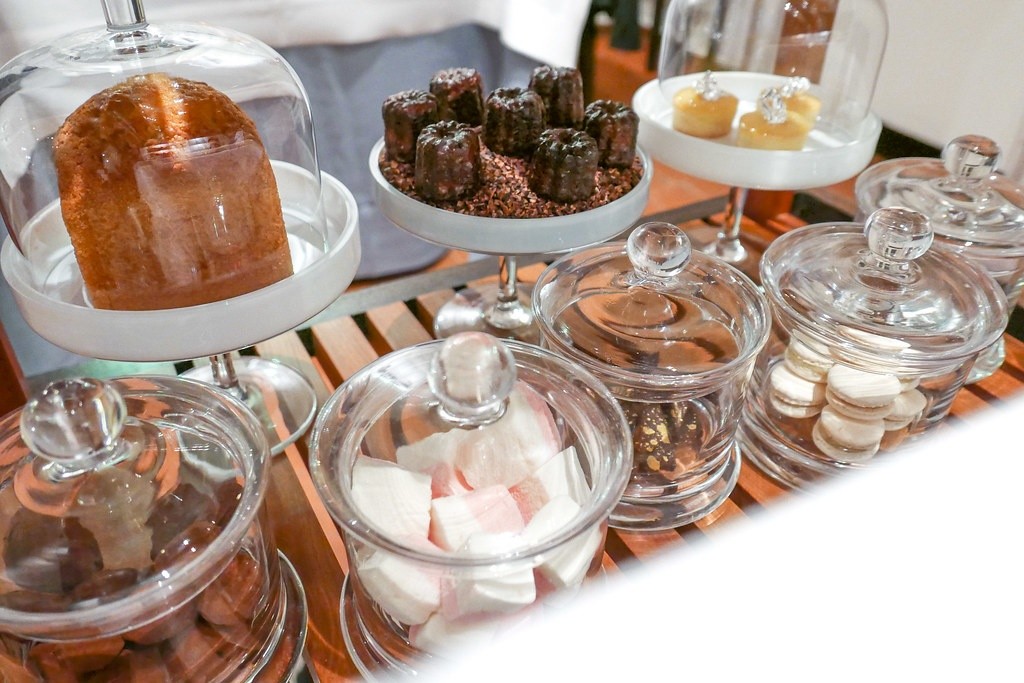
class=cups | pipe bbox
[738,203,1012,493]
[530,219,772,534]
[310,334,633,682]
[853,131,1024,385]
[0,372,311,682]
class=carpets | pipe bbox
[261,19,521,281]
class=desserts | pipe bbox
[2,64,926,683]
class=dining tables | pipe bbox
[1,179,1024,679]
